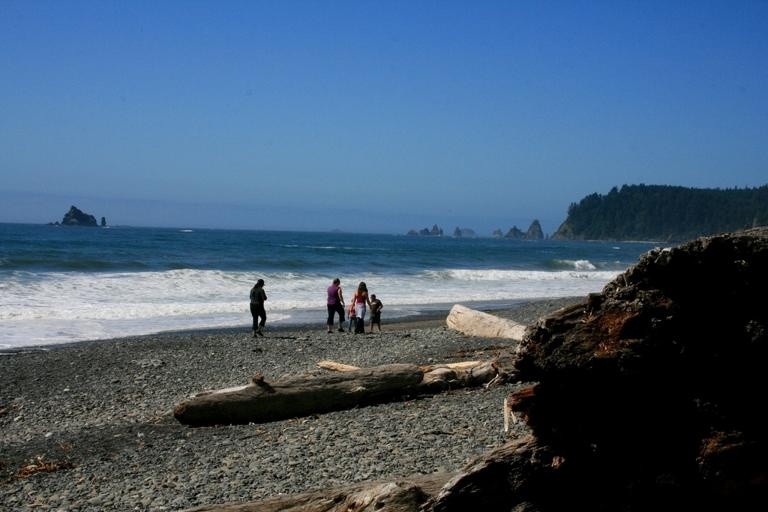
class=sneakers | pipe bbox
[328,330,333,333]
[252,330,263,338]
[338,328,345,332]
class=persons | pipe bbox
[347,298,357,334]
[367,294,383,333]
[352,282,370,334]
[326,277,345,334]
[249,279,267,338]
